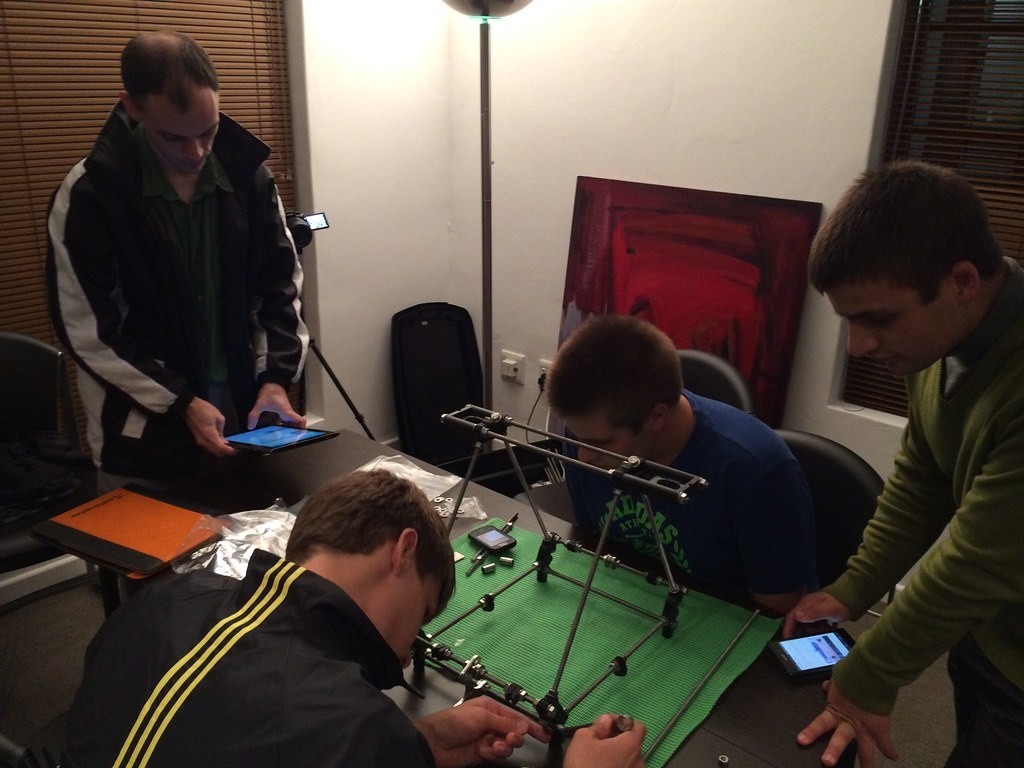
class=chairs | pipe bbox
[515,350,893,626]
[0,330,122,618]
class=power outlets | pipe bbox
[537,359,552,390]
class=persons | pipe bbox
[783,161,1024,768]
[44,31,310,517]
[546,314,818,614]
[57,469,647,768]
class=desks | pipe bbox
[101,427,856,768]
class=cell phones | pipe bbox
[468,525,517,553]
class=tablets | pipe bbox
[767,628,856,678]
[225,423,340,454]
